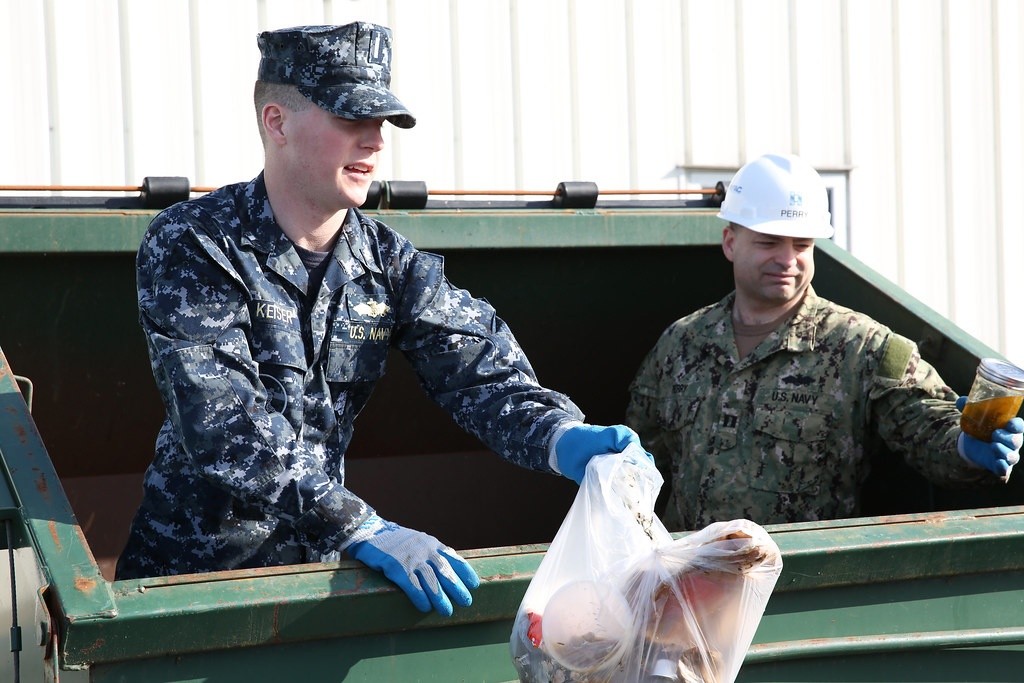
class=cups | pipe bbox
[960,358,1024,444]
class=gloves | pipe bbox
[337,507,481,616]
[546,420,656,486]
[957,395,1024,486]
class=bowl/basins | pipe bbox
[542,578,634,673]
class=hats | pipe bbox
[256,20,416,130]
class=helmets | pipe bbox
[716,151,834,239]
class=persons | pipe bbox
[623,152,1024,535]
[115,23,655,620]
